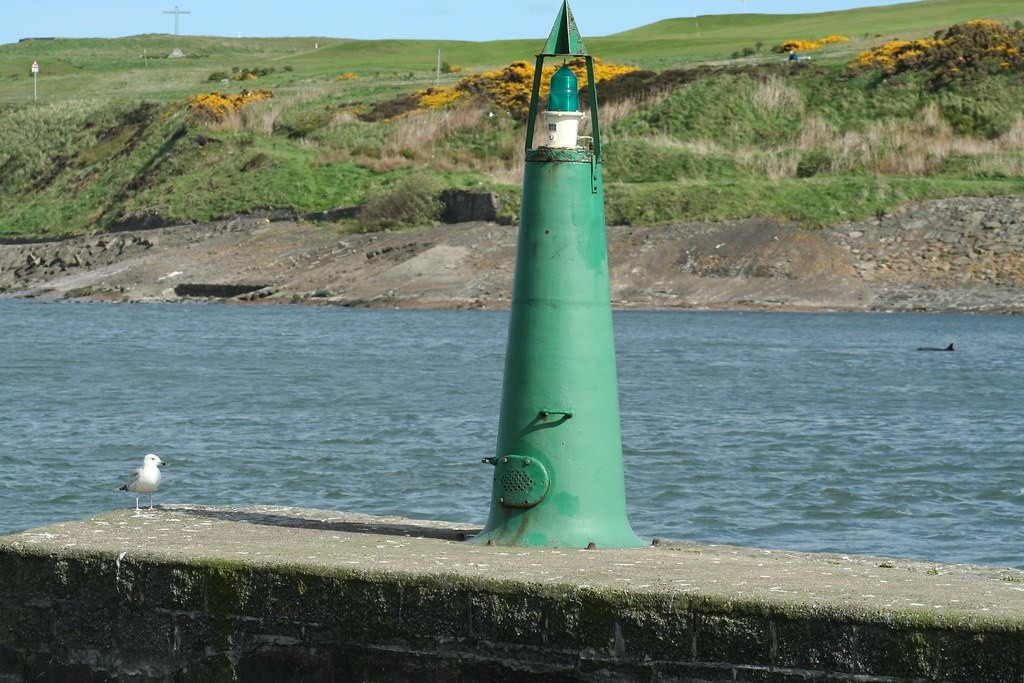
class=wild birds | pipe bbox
[117,453,166,511]
